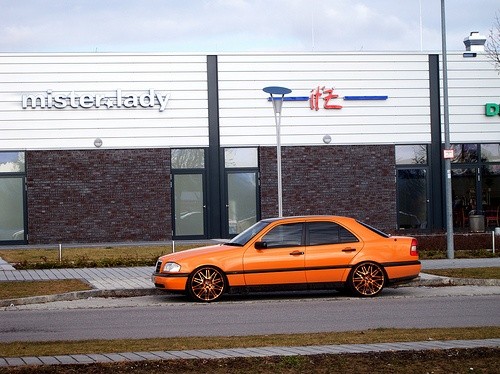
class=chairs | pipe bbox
[485,207,500,227]
[460,207,469,228]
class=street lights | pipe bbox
[263,86,290,218]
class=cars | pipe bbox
[173,210,256,235]
[151,214,422,302]
